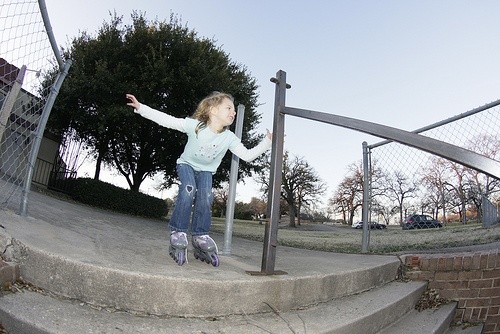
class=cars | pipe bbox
[351,221,386,229]
[402,215,442,229]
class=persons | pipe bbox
[126,91,287,267]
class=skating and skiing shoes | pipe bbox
[191,234,219,266]
[168,231,189,266]
